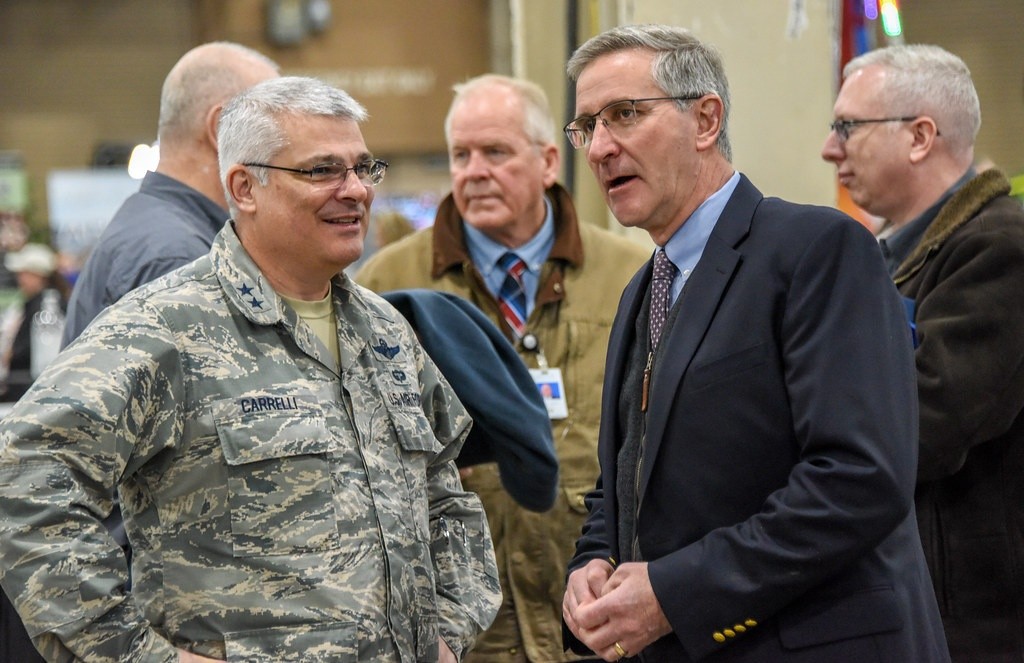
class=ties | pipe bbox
[496,252,528,342]
[650,249,679,351]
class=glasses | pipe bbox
[831,116,941,141]
[242,159,388,189]
[562,96,705,149]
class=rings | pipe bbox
[612,642,625,657]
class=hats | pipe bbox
[4,242,58,279]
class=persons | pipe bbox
[0,76,502,663]
[540,384,555,399]
[356,72,652,662]
[0,241,67,403]
[822,44,1024,663]
[59,41,280,354]
[562,23,950,663]
